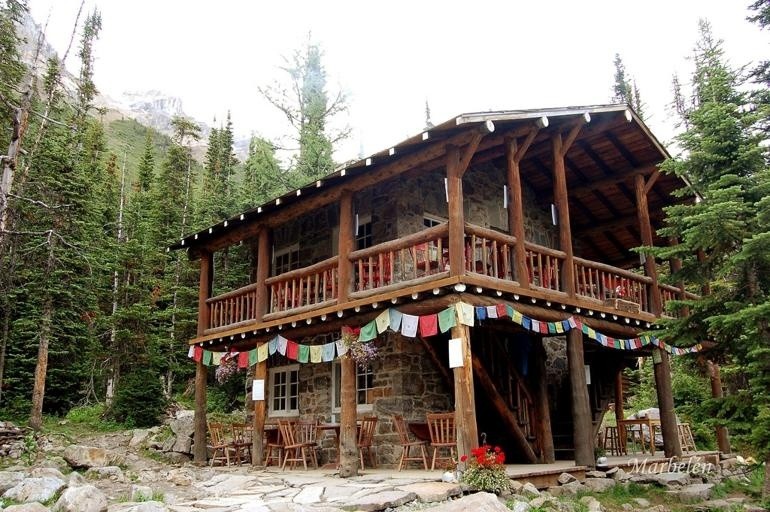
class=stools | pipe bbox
[603,418,698,457]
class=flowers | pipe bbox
[336,325,383,371]
[460,444,506,465]
[215,353,240,384]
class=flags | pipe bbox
[187,299,456,371]
[455,300,705,357]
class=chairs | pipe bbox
[475,241,647,310]
[274,242,438,310]
[207,411,457,472]
